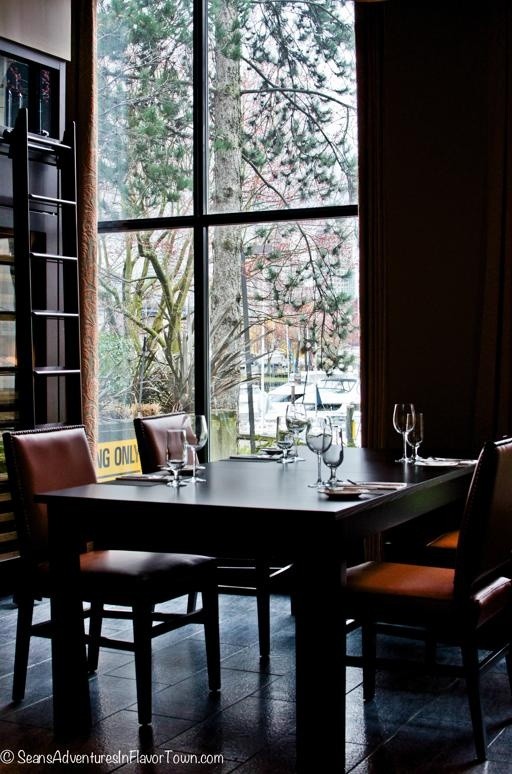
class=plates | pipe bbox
[161,464,206,475]
[317,487,371,499]
[260,448,293,455]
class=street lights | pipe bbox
[239,244,273,454]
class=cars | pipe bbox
[239,351,361,416]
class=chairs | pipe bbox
[344,431,512,765]
[2,410,298,725]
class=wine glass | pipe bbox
[166,430,187,488]
[181,414,208,483]
[275,402,308,463]
[306,417,344,488]
[393,403,425,463]
